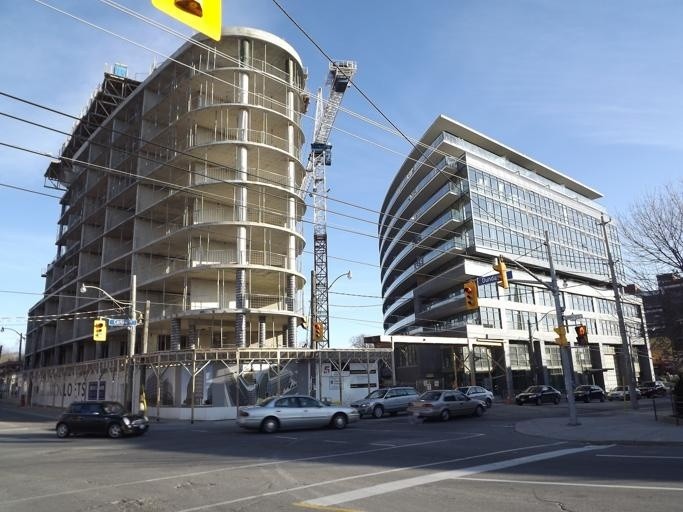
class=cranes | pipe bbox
[298,58,358,353]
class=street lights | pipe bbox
[311,271,352,397]
[80,284,143,412]
[1,328,24,408]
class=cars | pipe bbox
[607,386,641,401]
[515,385,561,405]
[568,384,605,403]
[408,389,487,420]
[56,400,150,438]
[456,386,493,408]
[639,381,667,396]
[238,394,360,434]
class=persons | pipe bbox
[448,384,454,390]
[466,383,469,387]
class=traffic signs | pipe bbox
[479,271,511,286]
[109,319,137,326]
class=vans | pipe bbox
[351,387,421,419]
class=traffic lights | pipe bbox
[492,261,509,289]
[463,282,478,310]
[576,325,588,344]
[312,323,323,340]
[94,320,107,341]
